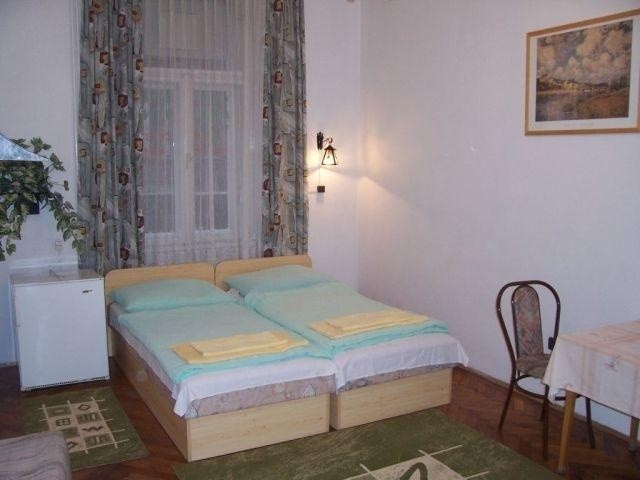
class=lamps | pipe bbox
[316,131,339,166]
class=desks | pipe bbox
[556,320,640,477]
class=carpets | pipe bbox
[20,383,152,473]
[170,407,567,480]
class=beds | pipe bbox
[103,261,336,463]
[215,256,469,432]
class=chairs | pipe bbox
[496,280,597,462]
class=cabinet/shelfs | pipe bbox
[8,267,110,392]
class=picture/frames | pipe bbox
[524,10,640,135]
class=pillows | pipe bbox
[112,275,238,312]
[225,263,335,294]
